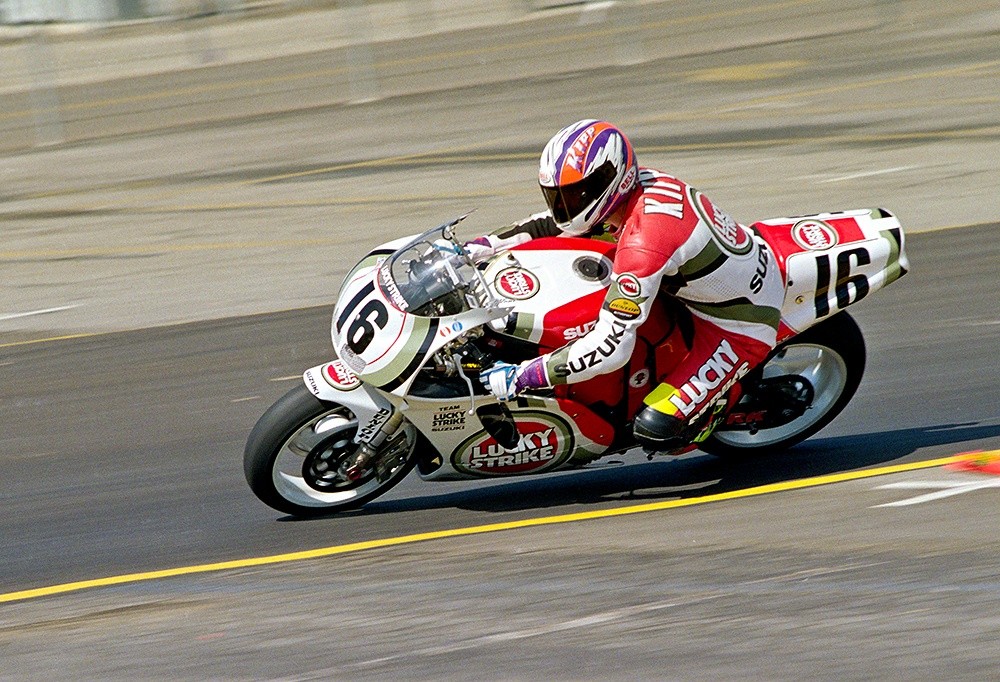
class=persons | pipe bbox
[421,118,785,457]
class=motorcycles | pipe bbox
[243,208,909,516]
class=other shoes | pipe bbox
[669,381,741,456]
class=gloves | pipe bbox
[416,235,496,269]
[479,353,555,403]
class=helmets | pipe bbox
[538,119,640,236]
